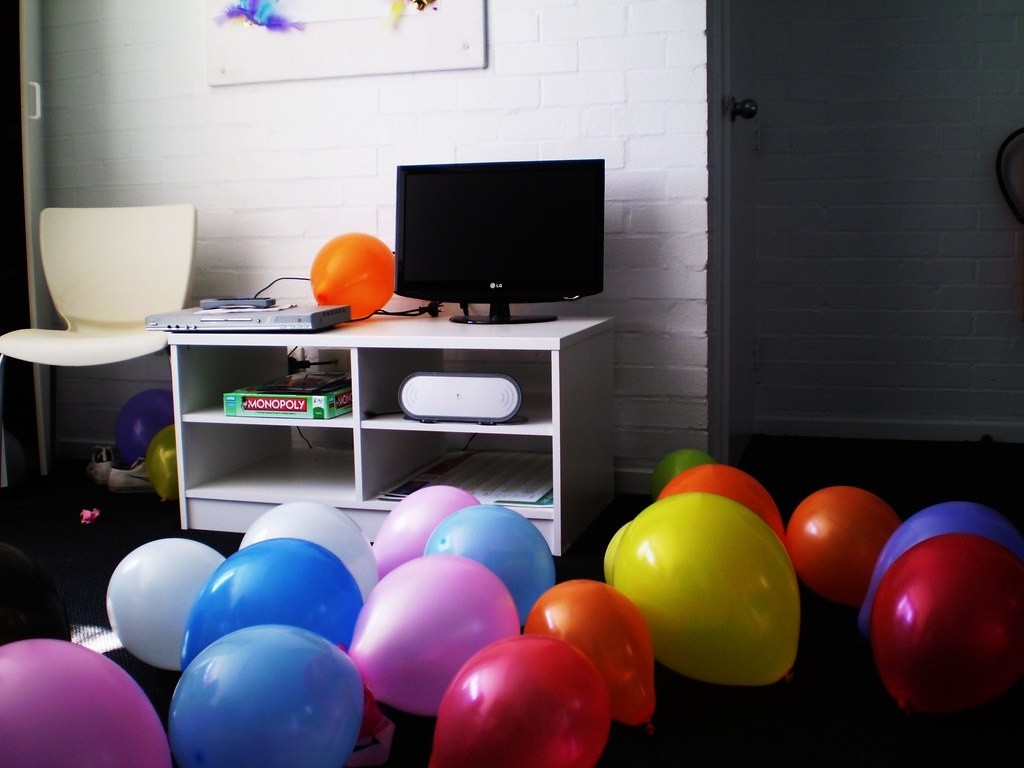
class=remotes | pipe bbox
[200,297,277,310]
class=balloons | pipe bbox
[146,424,179,501]
[311,233,394,320]
[114,389,175,466]
[0,448,1024,768]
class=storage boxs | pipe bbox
[223,374,351,419]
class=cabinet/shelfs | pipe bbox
[167,314,619,556]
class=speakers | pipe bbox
[398,372,522,426]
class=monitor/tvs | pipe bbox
[393,160,606,326]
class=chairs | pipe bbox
[0,203,198,485]
